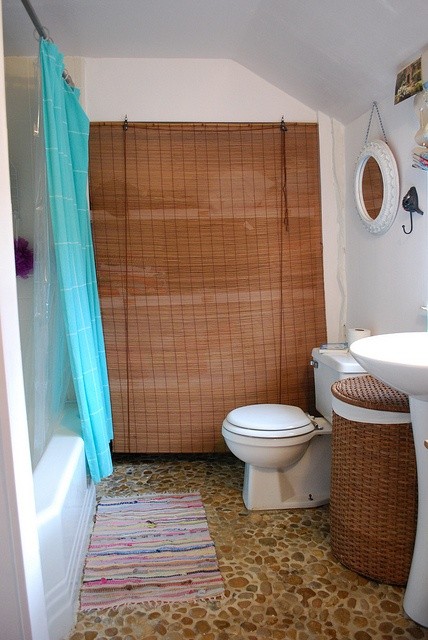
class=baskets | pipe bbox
[327,374,419,586]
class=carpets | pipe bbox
[81,489,225,613]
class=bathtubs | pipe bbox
[32,429,97,639]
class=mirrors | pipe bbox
[353,139,400,235]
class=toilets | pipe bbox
[221,346,367,511]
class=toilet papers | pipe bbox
[346,327,372,345]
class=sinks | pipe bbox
[348,332,427,396]
